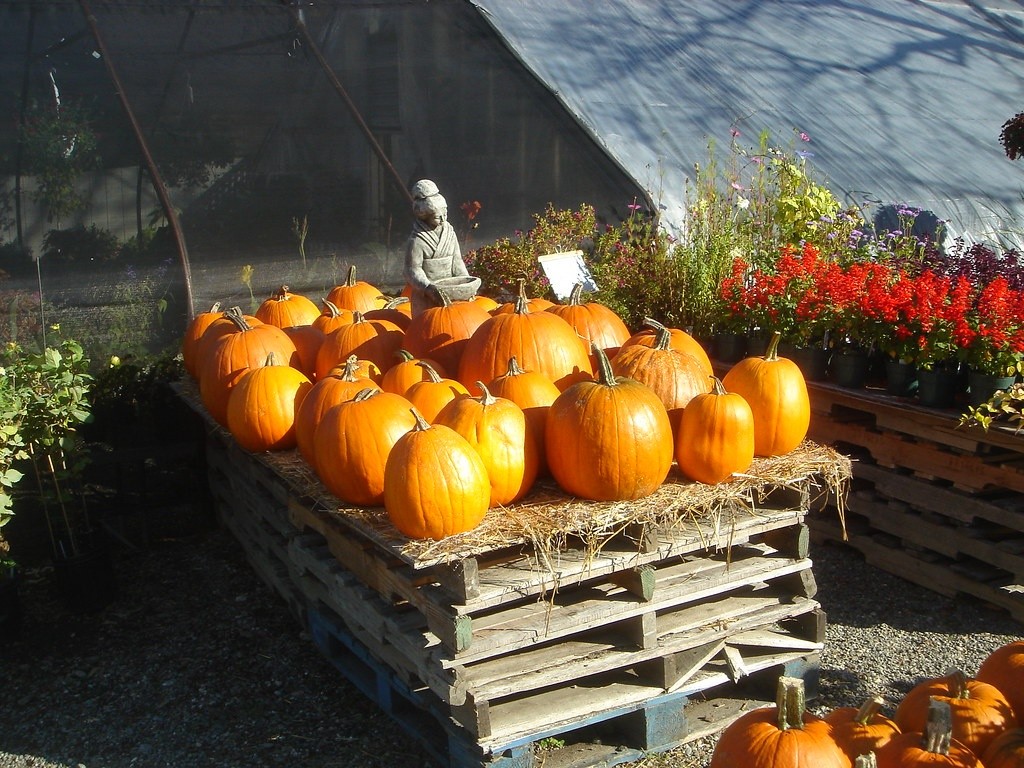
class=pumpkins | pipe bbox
[712,638,1024,768]
[183,265,811,540]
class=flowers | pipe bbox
[726,242,1023,378]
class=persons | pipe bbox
[402,177,483,321]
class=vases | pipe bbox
[874,361,912,396]
[717,334,746,362]
[747,339,771,358]
[796,349,826,380]
[970,373,1017,409]
[832,352,871,387]
[917,370,959,405]
